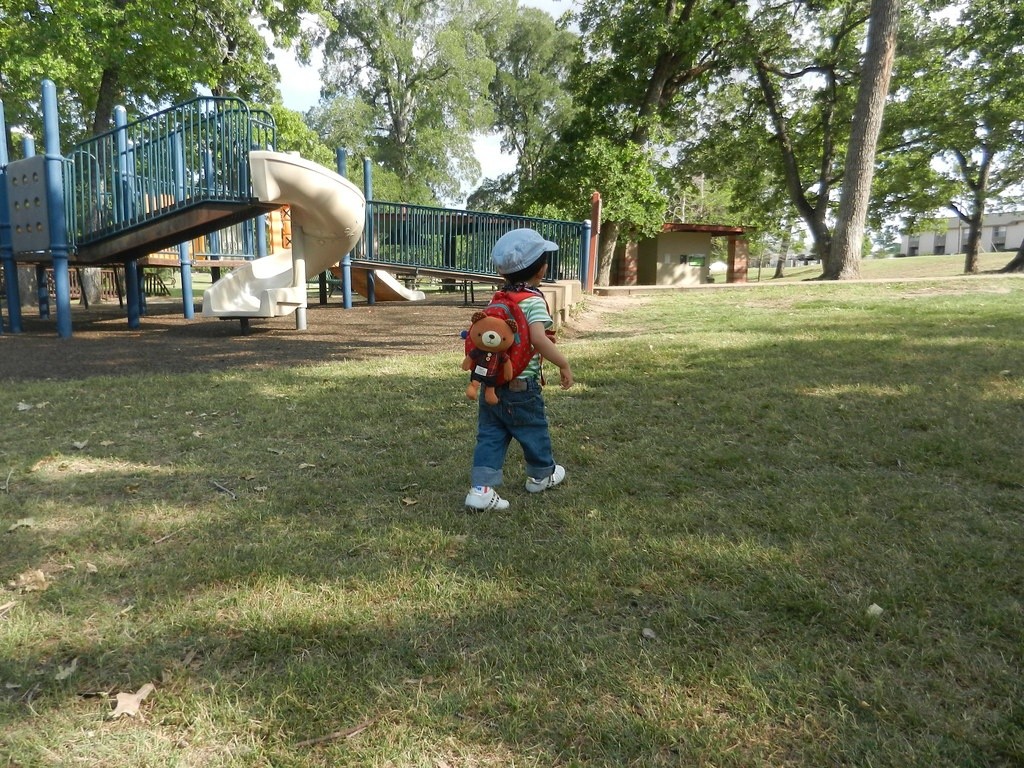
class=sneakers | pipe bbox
[464,487,509,511]
[525,465,565,493]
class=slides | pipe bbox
[203,151,365,318]
[330,260,426,301]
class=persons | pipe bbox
[464,228,573,510]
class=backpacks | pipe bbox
[459,292,554,386]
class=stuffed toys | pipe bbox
[461,312,518,406]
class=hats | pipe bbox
[491,230,559,275]
